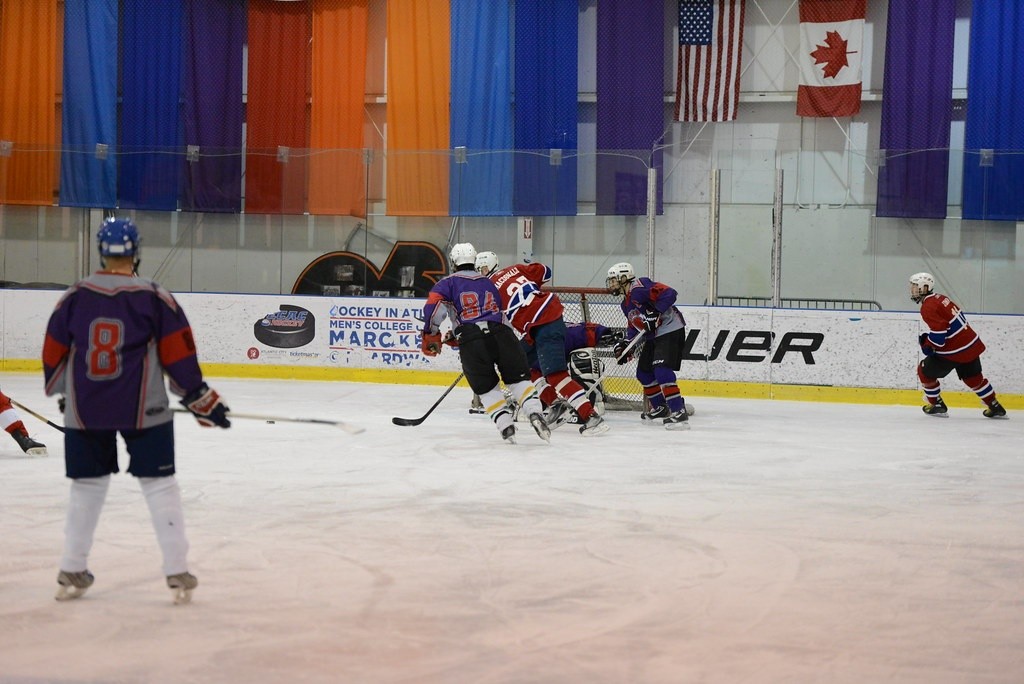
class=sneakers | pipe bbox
[55,570,94,600]
[11,429,49,458]
[983,404,1009,419]
[663,409,690,430]
[641,405,670,426]
[579,412,611,437]
[502,423,518,444]
[529,413,551,444]
[168,572,197,605]
[545,400,573,430]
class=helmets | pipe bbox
[449,243,477,271]
[475,251,499,276]
[909,273,935,304]
[605,263,635,296]
[97,216,139,257]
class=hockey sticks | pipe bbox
[392,372,466,427]
[545,326,647,430]
[2,395,68,434]
[170,408,367,436]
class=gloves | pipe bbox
[919,333,937,356]
[422,332,442,357]
[179,382,230,428]
[613,339,636,365]
[644,310,660,332]
[442,330,458,346]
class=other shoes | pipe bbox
[923,404,949,418]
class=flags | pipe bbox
[674,0,746,122]
[796,0,868,117]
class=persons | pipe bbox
[420,242,628,439]
[909,272,1006,418]
[0,386,47,454]
[41,217,231,589]
[605,262,689,425]
